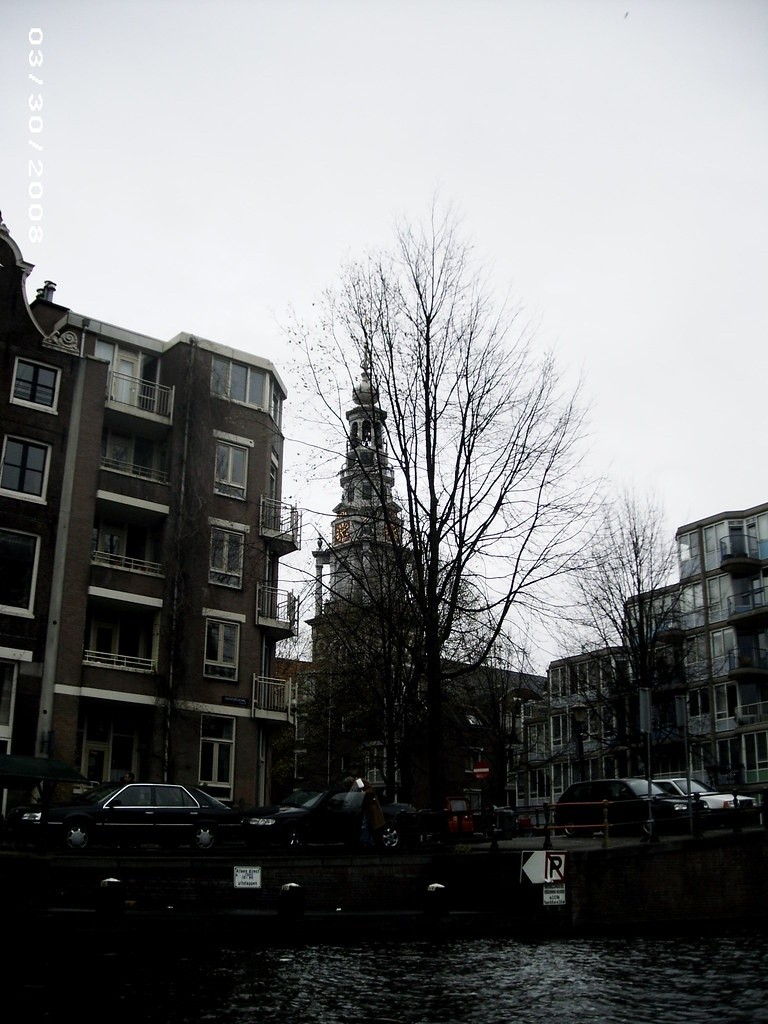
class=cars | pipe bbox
[0,778,250,855]
[553,777,712,840]
[237,784,420,856]
[648,776,758,829]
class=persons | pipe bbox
[123,770,136,782]
[361,791,389,858]
